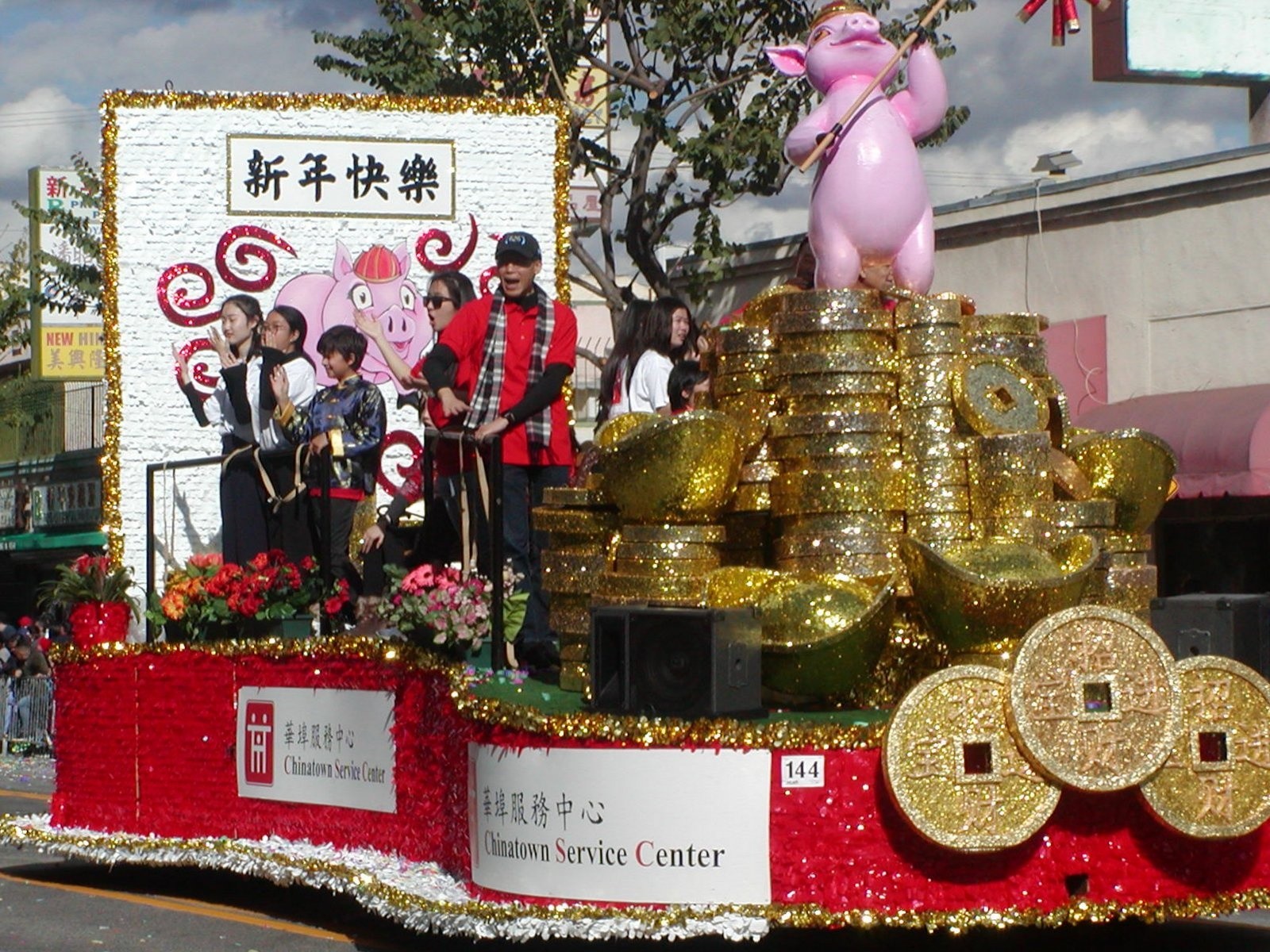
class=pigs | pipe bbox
[758,1,952,300]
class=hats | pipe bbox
[495,233,541,261]
[19,616,33,628]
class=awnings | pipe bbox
[0,524,108,552]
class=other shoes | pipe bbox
[514,641,562,672]
[23,744,57,761]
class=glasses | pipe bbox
[259,323,292,334]
[423,296,454,308]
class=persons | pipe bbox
[626,298,692,418]
[352,270,491,572]
[598,298,654,418]
[170,295,318,636]
[268,325,386,628]
[0,618,72,761]
[421,232,578,678]
[667,359,710,415]
